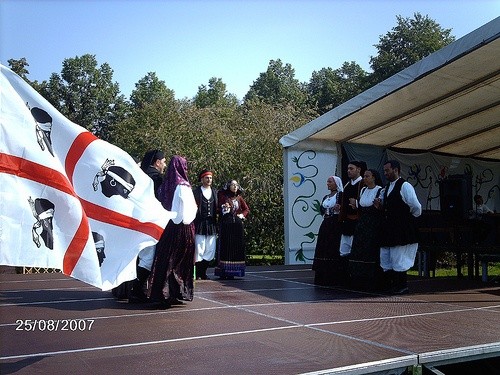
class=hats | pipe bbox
[350,160,363,168]
[140,149,165,169]
[198,170,213,182]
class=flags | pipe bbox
[0,64,177,292]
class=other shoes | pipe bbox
[169,300,187,305]
[201,275,210,279]
[196,276,201,280]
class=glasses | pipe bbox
[230,184,238,186]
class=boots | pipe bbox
[128,266,153,304]
[383,270,408,294]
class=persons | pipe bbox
[312,160,422,296]
[108,146,250,309]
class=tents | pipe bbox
[278,16,500,266]
